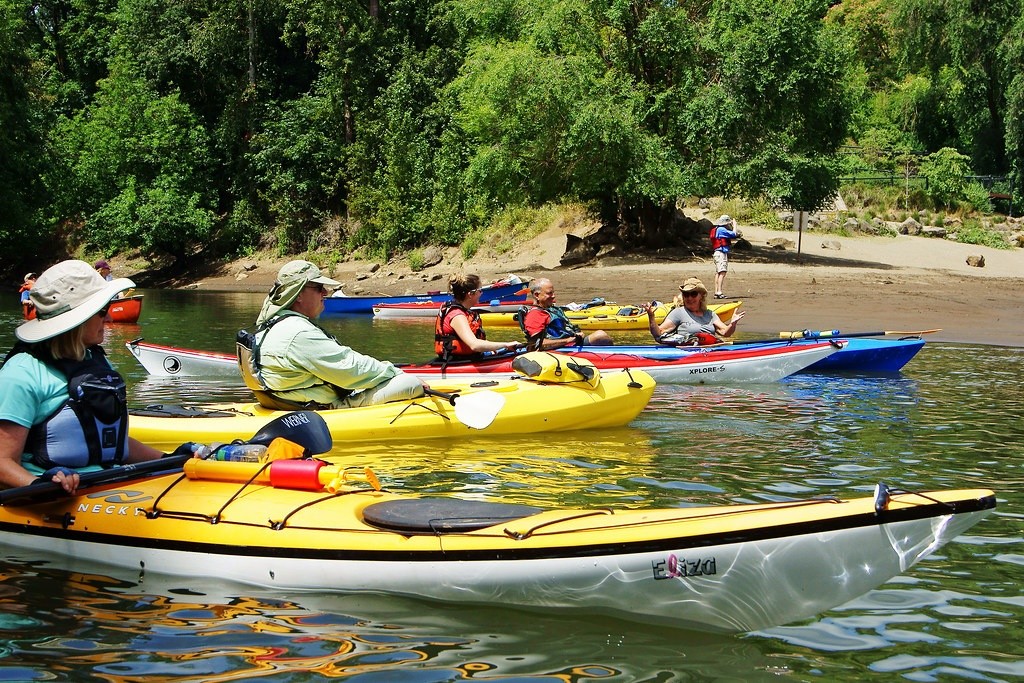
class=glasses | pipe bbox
[683,290,699,298]
[304,283,324,293]
[95,305,111,318]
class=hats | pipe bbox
[255,260,341,326]
[679,278,708,297]
[714,215,732,225]
[14,260,137,344]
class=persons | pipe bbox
[1,259,213,497]
[19,272,39,308]
[524,276,614,350]
[642,276,747,347]
[711,213,738,299]
[433,272,523,356]
[93,259,125,301]
[253,259,431,411]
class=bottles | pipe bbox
[195,443,268,463]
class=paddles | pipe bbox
[479,288,530,304]
[512,338,541,349]
[125,288,136,298]
[674,328,944,350]
[425,387,506,430]
[552,324,578,337]
[28,410,333,504]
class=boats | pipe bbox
[372,300,535,317]
[487,340,927,372]
[324,281,529,313]
[128,368,657,453]
[124,338,849,386]
[0,471,996,636]
[568,300,743,330]
[478,301,678,327]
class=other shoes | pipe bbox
[714,292,727,299]
